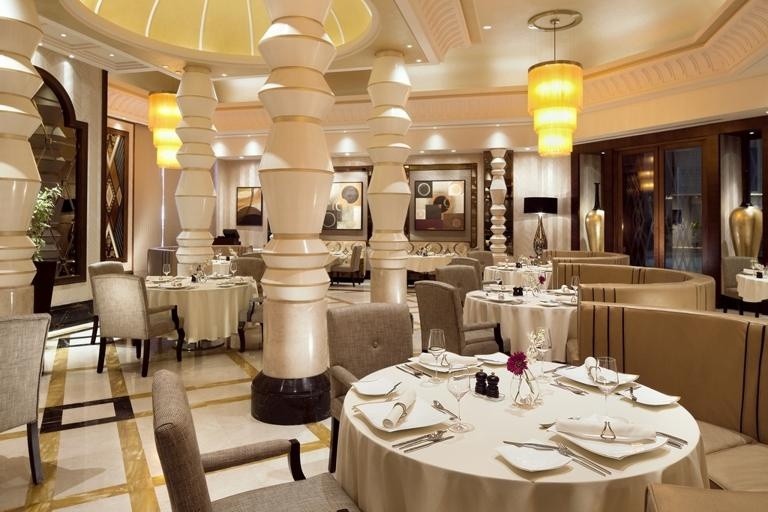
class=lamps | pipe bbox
[524,197,558,259]
[523,8,586,159]
[148,88,184,171]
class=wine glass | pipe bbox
[215,250,219,262]
[218,248,223,261]
[494,271,579,296]
[230,261,237,278]
[429,327,619,433]
[751,256,758,278]
[447,367,472,432]
[429,329,446,383]
[163,264,171,278]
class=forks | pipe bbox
[551,384,583,394]
[556,440,612,476]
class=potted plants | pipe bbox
[23,184,65,312]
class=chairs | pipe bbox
[225,256,263,353]
[0,312,55,485]
[92,272,187,377]
[148,369,357,511]
[87,260,125,344]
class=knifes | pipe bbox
[433,400,458,420]
[404,436,455,453]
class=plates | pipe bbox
[554,362,640,387]
[490,261,552,272]
[146,274,247,289]
[498,437,572,472]
[596,356,618,406]
[407,351,485,371]
[548,417,668,460]
[474,352,511,365]
[355,398,449,432]
[615,381,681,406]
[350,374,400,396]
[472,287,578,306]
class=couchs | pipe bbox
[146,244,254,277]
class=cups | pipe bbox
[510,367,539,406]
